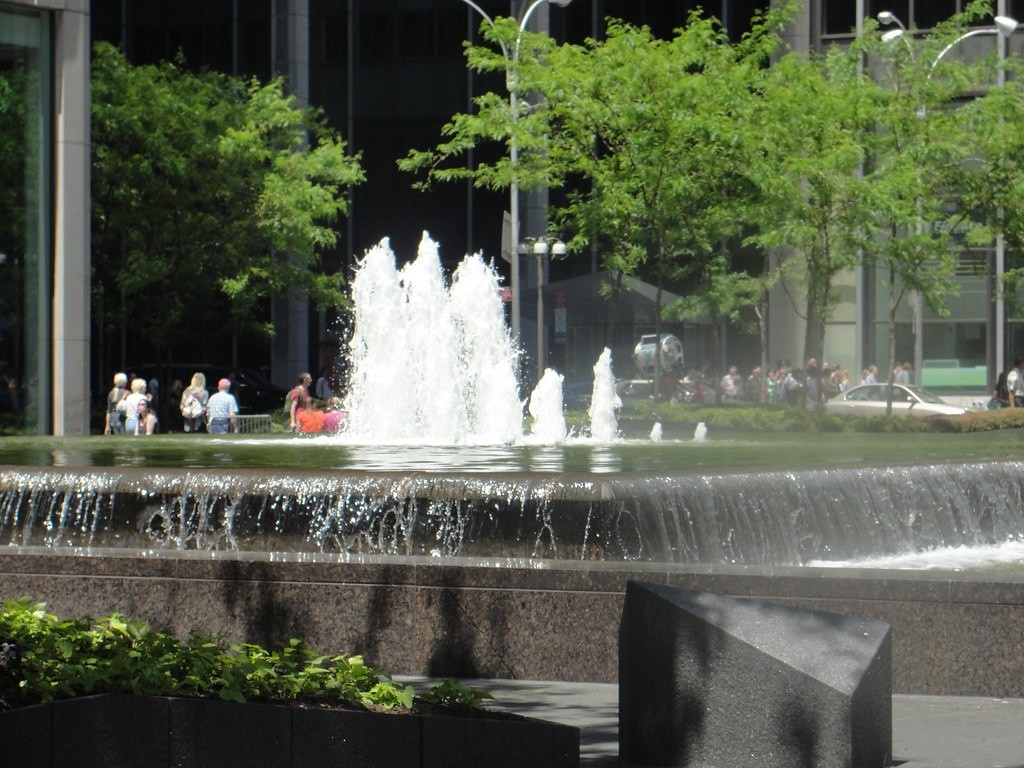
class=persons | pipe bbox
[660,358,878,406]
[992,357,1024,408]
[893,361,911,390]
[285,367,348,431]
[103,372,240,436]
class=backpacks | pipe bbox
[182,388,203,419]
[284,387,303,418]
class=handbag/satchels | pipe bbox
[988,390,1002,410]
[116,390,129,415]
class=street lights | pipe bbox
[875,11,1018,388]
[517,236,568,390]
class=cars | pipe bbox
[823,381,973,418]
